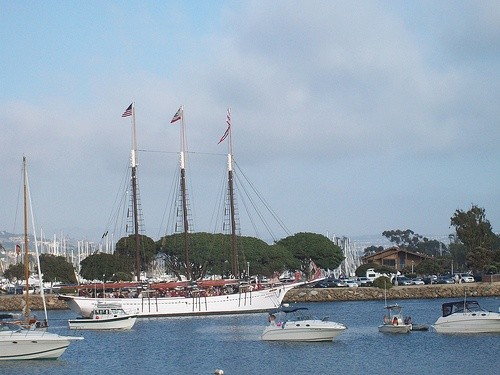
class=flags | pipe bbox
[122,103,134,118]
[170,105,182,123]
[216,108,231,144]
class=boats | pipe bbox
[412,323,429,331]
[4,322,48,331]
[378,303,412,333]
[68,304,138,330]
[431,287,500,333]
[259,306,346,342]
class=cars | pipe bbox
[0,272,61,295]
[333,268,474,287]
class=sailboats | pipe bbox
[0,156,85,360]
[55,102,328,318]
